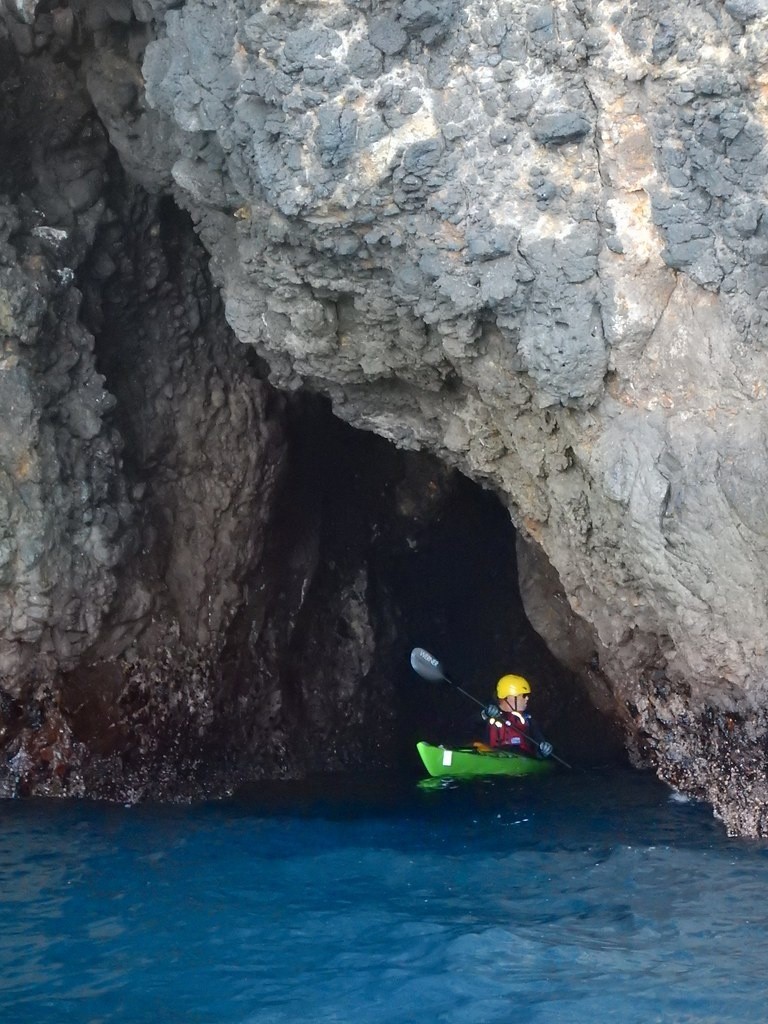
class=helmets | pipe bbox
[497,675,531,699]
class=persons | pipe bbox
[488,674,553,762]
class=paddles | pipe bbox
[411,647,572,770]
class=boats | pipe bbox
[416,742,566,786]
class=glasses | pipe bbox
[522,693,531,699]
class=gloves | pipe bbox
[539,742,554,756]
[485,704,503,717]
